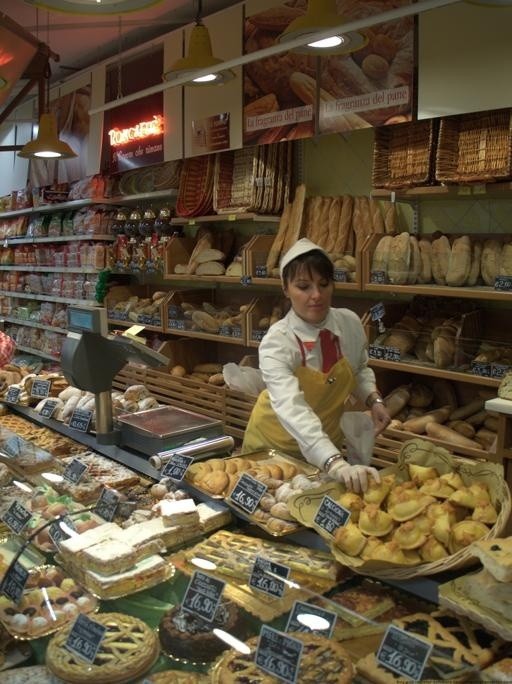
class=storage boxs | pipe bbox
[153,141,293,214]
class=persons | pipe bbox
[238,238,393,494]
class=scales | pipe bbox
[60,304,225,456]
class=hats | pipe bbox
[279,238,332,294]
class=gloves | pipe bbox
[328,460,382,493]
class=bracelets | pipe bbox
[370,398,388,409]
[325,454,341,473]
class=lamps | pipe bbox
[16,0,79,162]
[160,1,375,89]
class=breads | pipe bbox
[0,182,512,683]
[243,0,416,149]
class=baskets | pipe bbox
[333,455,512,580]
[119,140,292,217]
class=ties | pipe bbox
[318,330,338,373]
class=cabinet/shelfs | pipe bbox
[0,172,512,469]
